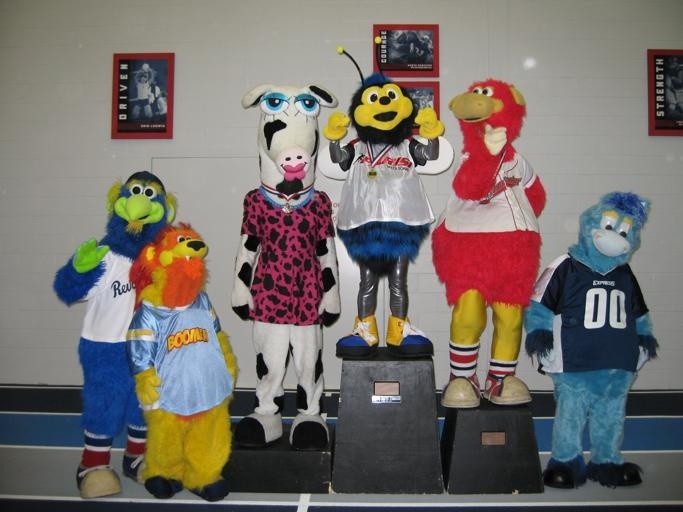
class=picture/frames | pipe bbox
[393,79,441,138]
[647,49,683,137]
[110,49,176,139]
[372,22,439,79]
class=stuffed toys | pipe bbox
[48,167,180,500]
[317,71,456,360]
[524,189,660,487]
[424,79,546,408]
[124,220,241,504]
[229,81,341,450]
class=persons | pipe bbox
[386,30,433,108]
[128,62,166,123]
